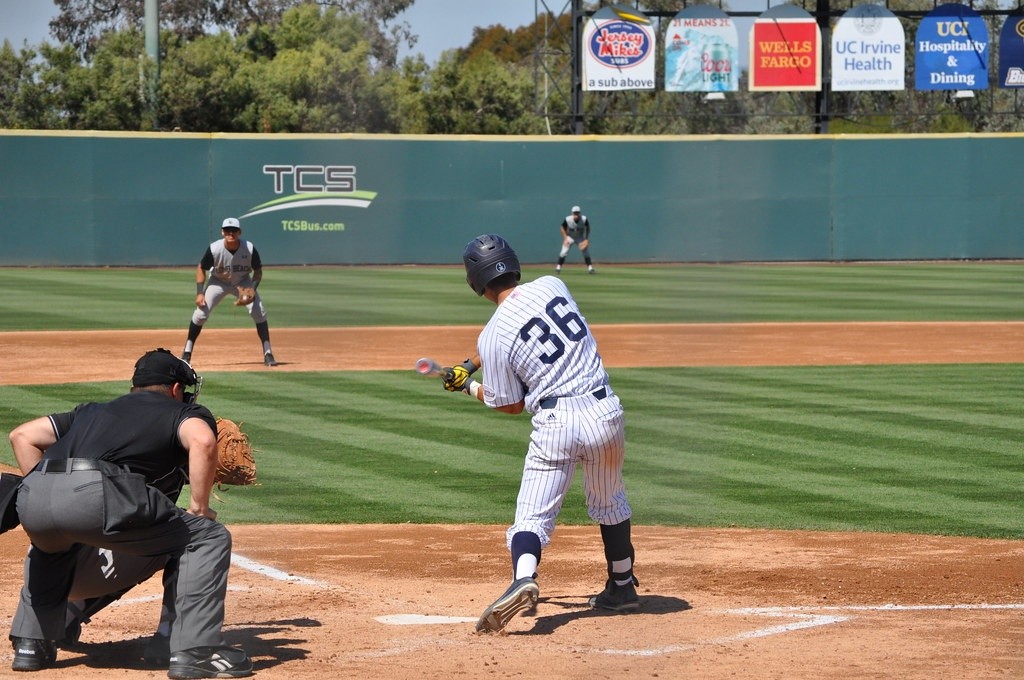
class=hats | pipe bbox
[572,206,580,213]
[220,217,241,230]
[134,350,200,387]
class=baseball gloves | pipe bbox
[579,239,590,251]
[234,287,258,307]
[214,415,258,487]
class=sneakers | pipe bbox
[588,577,640,612]
[141,630,254,679]
[12,624,82,671]
[476,572,540,633]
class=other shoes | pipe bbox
[555,268,594,274]
[181,353,191,361]
[264,353,275,365]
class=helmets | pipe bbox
[463,234,521,296]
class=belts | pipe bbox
[35,457,101,473]
[539,387,607,409]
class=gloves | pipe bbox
[442,358,478,395]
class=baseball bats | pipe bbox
[416,359,453,381]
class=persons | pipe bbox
[554,206,595,274]
[8,348,254,679]
[182,218,276,366]
[443,234,641,635]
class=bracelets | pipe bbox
[196,282,204,294]
[251,281,259,290]
[469,380,481,398]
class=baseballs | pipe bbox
[242,295,250,302]
